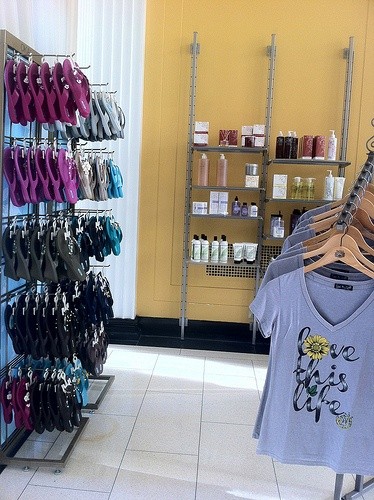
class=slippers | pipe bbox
[0,59,125,434]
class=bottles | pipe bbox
[241,201,258,217]
[198,153,209,185]
[269,214,284,238]
[216,154,227,186]
[191,234,228,264]
